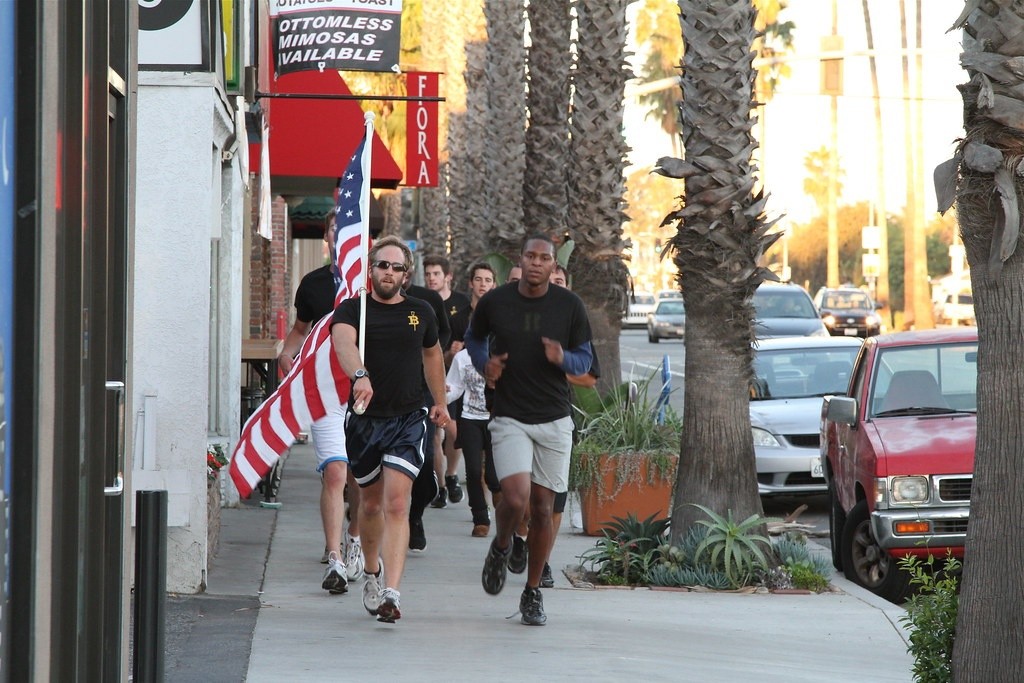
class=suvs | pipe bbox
[813,285,883,336]
[622,292,656,328]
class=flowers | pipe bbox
[207,444,229,481]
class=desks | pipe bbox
[240,336,282,398]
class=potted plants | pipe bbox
[567,360,683,537]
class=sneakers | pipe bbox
[430,486,448,509]
[408,517,427,553]
[507,533,528,574]
[321,533,343,564]
[538,561,554,588]
[505,588,547,626]
[373,586,401,624]
[482,537,512,595]
[473,523,490,537]
[341,523,364,582]
[359,556,383,616]
[445,471,464,504]
[321,550,349,594]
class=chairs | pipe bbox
[812,362,852,395]
[878,369,939,410]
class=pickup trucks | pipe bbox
[818,325,977,601]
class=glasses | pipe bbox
[328,224,338,232]
[370,260,407,273]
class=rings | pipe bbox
[444,423,446,426]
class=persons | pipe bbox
[403,273,452,553]
[447,347,500,538]
[328,236,451,624]
[508,265,601,587]
[449,263,496,519]
[279,208,365,594]
[463,234,593,626]
[422,256,470,509]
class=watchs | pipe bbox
[355,369,369,378]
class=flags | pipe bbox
[229,134,367,502]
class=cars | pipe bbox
[659,291,684,302]
[748,334,894,512]
[647,299,685,344]
[751,282,833,384]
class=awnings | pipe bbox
[249,69,404,191]
